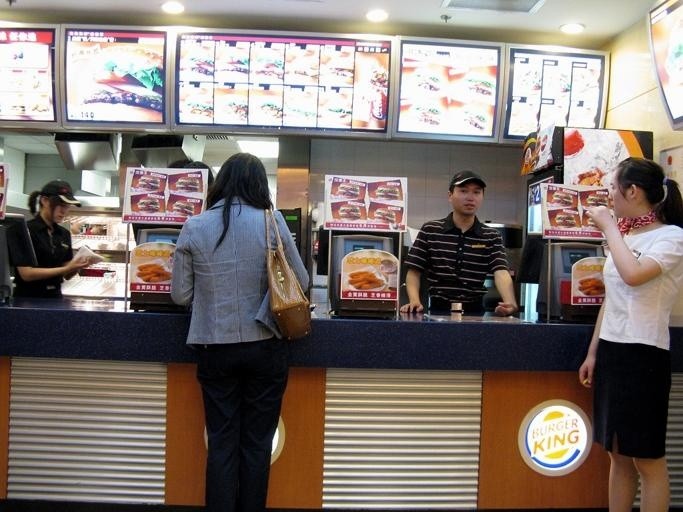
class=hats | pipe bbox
[449,170,487,188]
[42,179,81,208]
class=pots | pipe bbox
[78,269,111,277]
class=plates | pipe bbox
[136,263,170,283]
[349,270,386,291]
[576,276,603,296]
[385,267,395,273]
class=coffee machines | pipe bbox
[483,220,523,288]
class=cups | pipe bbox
[97,242,108,250]
[380,259,392,271]
[369,81,388,121]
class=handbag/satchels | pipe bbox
[265,208,313,340]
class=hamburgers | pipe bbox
[337,183,400,223]
[137,175,200,216]
[552,191,608,227]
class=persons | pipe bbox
[576,155,682,511]
[168,152,314,512]
[4,178,92,300]
[399,169,520,318]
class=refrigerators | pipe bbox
[519,129,655,323]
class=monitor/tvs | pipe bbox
[146,231,180,246]
[561,246,598,273]
[344,238,385,256]
